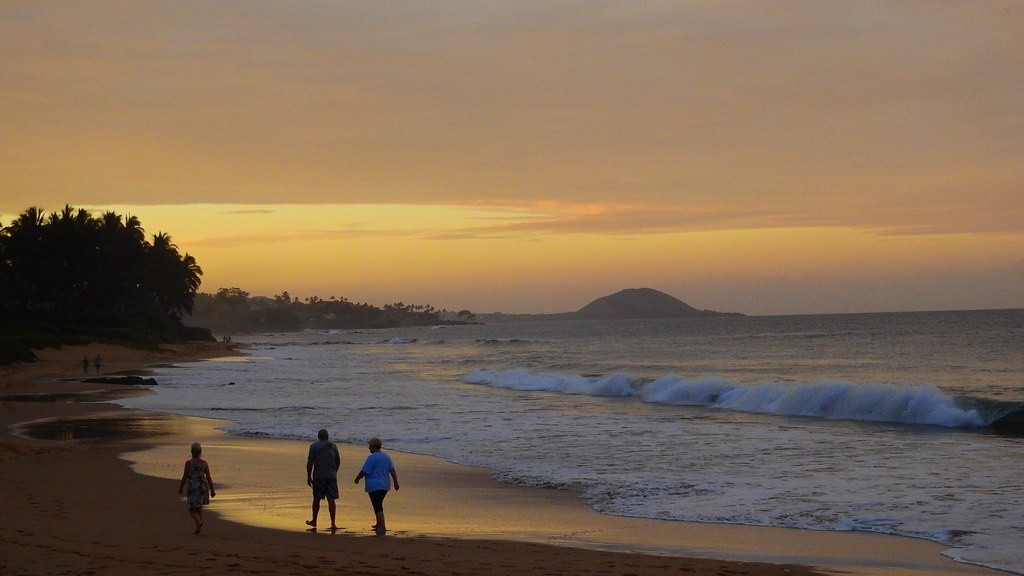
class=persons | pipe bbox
[95,354,102,372]
[83,356,89,374]
[354,438,399,531]
[179,443,216,535]
[223,335,231,342]
[306,429,340,529]
